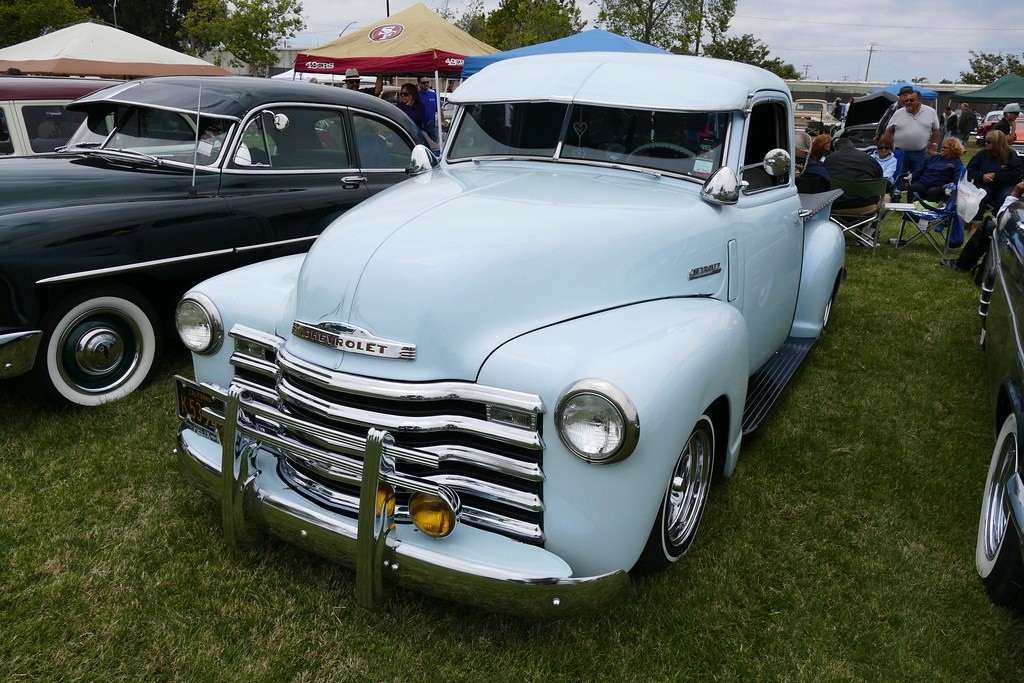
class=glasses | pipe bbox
[400,92,410,97]
[879,146,889,150]
[942,146,950,149]
[349,80,360,84]
[1008,112,1019,115]
[905,99,914,102]
[984,140,991,144]
[419,81,429,84]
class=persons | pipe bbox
[965,129,1024,222]
[823,137,884,241]
[883,89,941,203]
[416,75,445,143]
[794,131,832,195]
[395,82,428,155]
[939,106,958,151]
[992,102,1024,145]
[811,131,834,165]
[830,98,842,121]
[936,182,1024,272]
[326,68,380,134]
[869,134,897,192]
[957,103,979,144]
[905,136,967,203]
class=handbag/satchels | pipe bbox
[949,214,964,248]
[955,169,987,223]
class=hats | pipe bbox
[342,68,363,80]
[897,86,913,96]
[1004,103,1020,112]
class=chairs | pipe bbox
[895,164,967,257]
[32,138,70,152]
[829,176,889,255]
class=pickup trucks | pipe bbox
[791,98,841,128]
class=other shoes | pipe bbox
[940,257,959,269]
[855,240,880,247]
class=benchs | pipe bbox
[288,148,410,168]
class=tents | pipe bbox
[866,81,938,114]
[292,1,504,157]
[452,27,673,121]
[0,22,236,79]
[843,97,853,120]
[270,67,378,88]
[947,70,1024,107]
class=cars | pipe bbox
[964,200,1024,603]
[0,74,221,160]
[2,76,440,403]
[971,111,1024,144]
[164,46,852,617]
